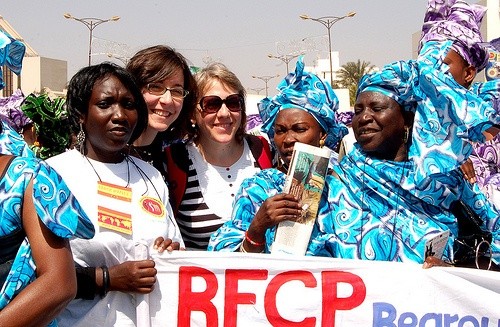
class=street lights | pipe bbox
[63,12,121,74]
[268,51,305,79]
[249,86,269,97]
[251,72,280,100]
[299,10,357,89]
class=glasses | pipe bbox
[143,82,190,99]
[197,93,245,113]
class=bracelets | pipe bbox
[240,230,265,255]
[98,264,110,297]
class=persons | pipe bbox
[159,60,273,250]
[0,119,96,326]
[323,59,457,268]
[123,43,196,220]
[206,56,350,250]
[45,60,184,327]
[413,1,500,266]
